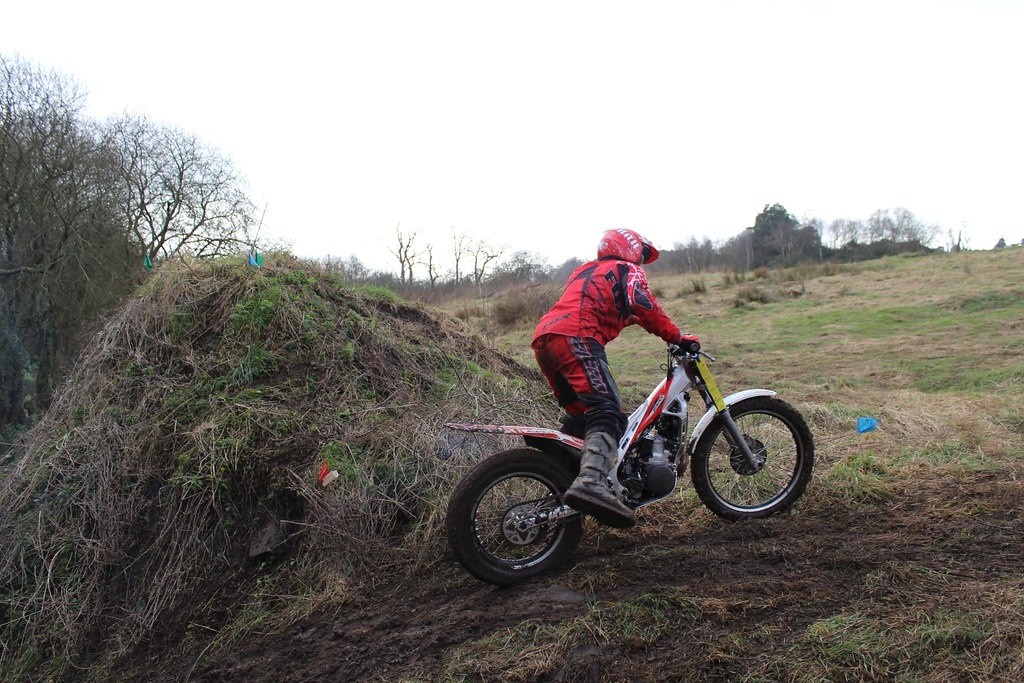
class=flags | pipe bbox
[857,417,878,434]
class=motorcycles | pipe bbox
[443,334,816,587]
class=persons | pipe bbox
[530,228,702,529]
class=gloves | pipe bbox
[634,316,652,333]
[676,331,700,356]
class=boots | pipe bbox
[562,431,636,528]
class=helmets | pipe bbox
[598,229,659,264]
